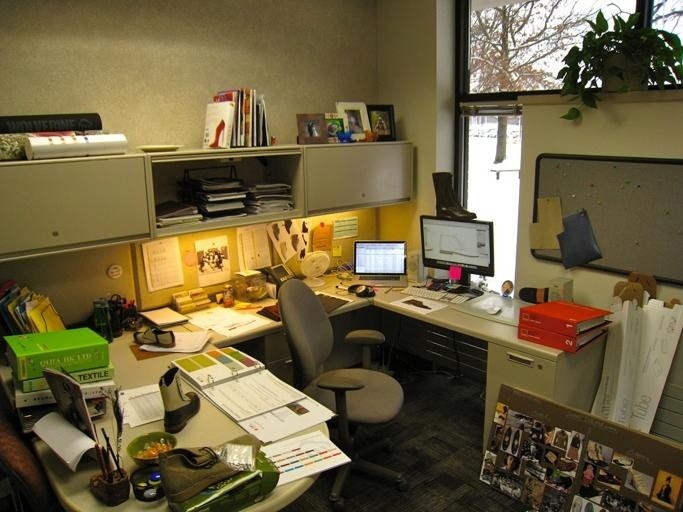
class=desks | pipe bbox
[348,253,610,461]
[175,260,373,351]
[1,306,332,510]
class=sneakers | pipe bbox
[571,497,583,512]
[585,502,595,512]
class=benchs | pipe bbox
[491,169,521,178]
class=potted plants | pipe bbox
[557,9,683,120]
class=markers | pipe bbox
[336,286,349,291]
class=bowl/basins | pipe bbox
[128,432,177,468]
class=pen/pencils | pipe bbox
[385,287,393,294]
[95,443,108,479]
[101,428,123,478]
[102,446,114,482]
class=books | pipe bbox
[1,326,115,472]
[516,299,614,354]
[169,345,351,483]
[156,167,294,229]
[202,86,269,149]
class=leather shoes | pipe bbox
[154,432,264,504]
[612,456,635,467]
[511,429,521,455]
[501,425,512,451]
[131,326,177,349]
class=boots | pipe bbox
[157,364,202,435]
[430,170,479,221]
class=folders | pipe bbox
[518,324,608,353]
[519,301,614,337]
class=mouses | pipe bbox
[486,304,503,316]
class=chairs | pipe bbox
[277,279,410,509]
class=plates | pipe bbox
[137,144,183,152]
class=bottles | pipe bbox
[92,296,113,343]
[223,284,234,307]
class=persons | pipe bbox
[656,476,675,504]
[498,452,520,476]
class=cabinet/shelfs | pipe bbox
[1,139,416,265]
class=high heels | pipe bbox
[208,119,227,149]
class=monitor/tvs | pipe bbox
[417,213,496,296]
[350,238,409,288]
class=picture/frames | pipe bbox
[325,113,349,143]
[335,101,373,141]
[367,102,396,142]
[296,111,326,145]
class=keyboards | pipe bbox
[402,284,468,305]
